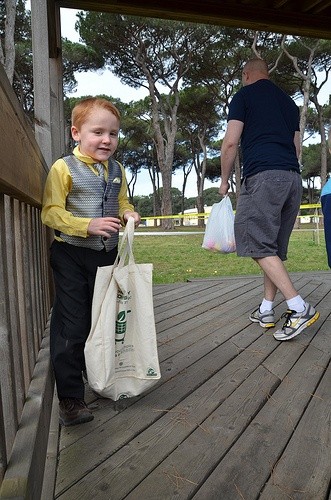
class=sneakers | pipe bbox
[273,302,320,341]
[59,398,94,427]
[249,303,275,327]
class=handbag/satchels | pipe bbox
[83,217,162,402]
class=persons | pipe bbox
[40,98,161,425]
[321,175,331,270]
[219,58,319,341]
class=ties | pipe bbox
[92,162,107,193]
